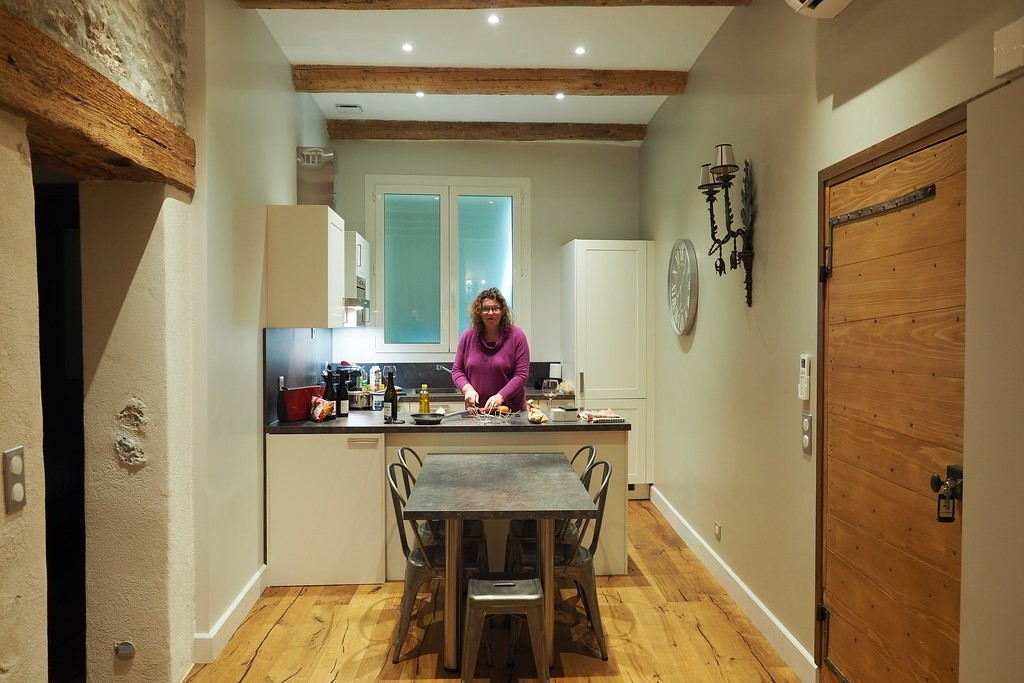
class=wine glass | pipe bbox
[542,379,560,420]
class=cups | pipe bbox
[383,365,397,379]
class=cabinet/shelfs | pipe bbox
[263,433,629,588]
[260,204,347,329]
[344,229,371,326]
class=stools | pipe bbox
[458,577,553,682]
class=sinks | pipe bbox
[411,388,462,395]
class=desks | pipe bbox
[403,453,599,671]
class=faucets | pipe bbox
[436,365,462,395]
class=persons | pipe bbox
[450,288,530,412]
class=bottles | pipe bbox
[336,373,349,417]
[362,373,368,391]
[322,370,336,420]
[419,384,430,413]
[383,371,397,421]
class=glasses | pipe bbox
[481,306,502,313]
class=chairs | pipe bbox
[388,462,493,665]
[506,460,614,670]
[397,446,484,544]
[503,443,597,595]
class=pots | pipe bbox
[410,409,471,423]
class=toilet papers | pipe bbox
[549,364,562,378]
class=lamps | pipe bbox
[695,144,754,307]
